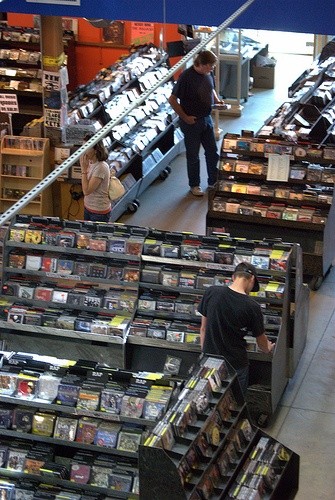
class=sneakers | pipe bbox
[190,185,204,195]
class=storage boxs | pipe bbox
[252,61,274,88]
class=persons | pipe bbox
[196,261,277,404]
[167,51,225,196]
[79,133,113,223]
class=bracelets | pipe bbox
[81,172,88,175]
[179,112,185,119]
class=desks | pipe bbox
[211,43,267,103]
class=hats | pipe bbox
[236,262,260,292]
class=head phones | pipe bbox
[69,183,84,200]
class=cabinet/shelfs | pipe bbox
[0,21,335,499]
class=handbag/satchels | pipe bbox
[110,176,126,201]
